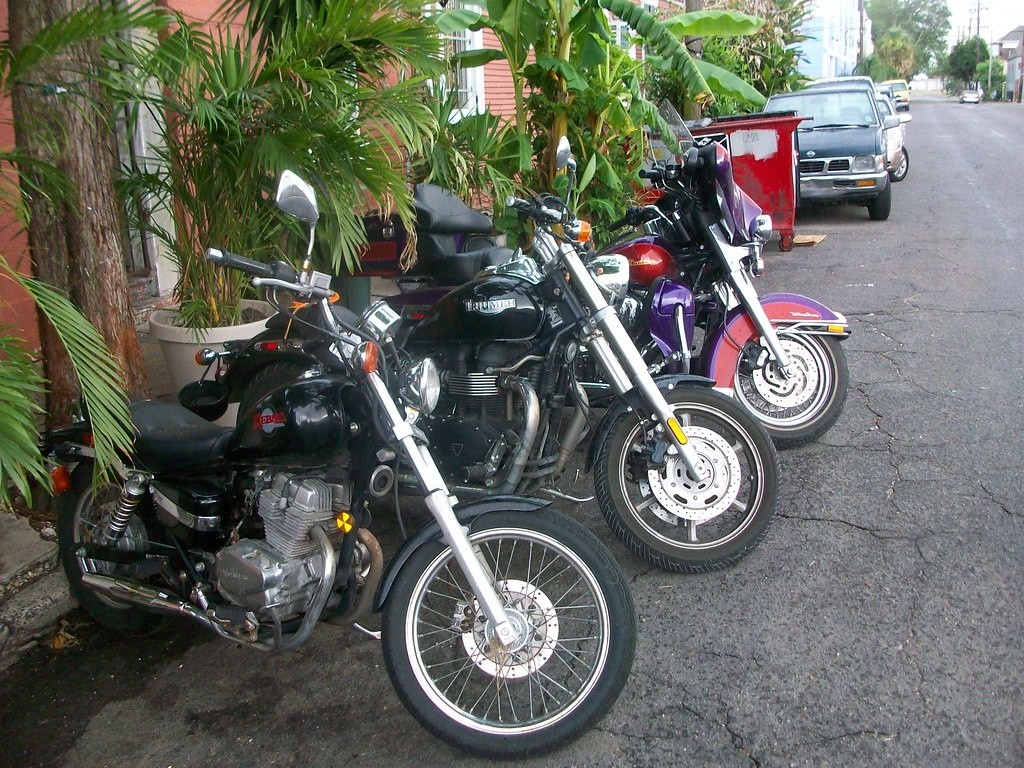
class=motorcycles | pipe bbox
[40,166,640,762]
[195,133,785,574]
[335,100,854,453]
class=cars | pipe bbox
[959,88,980,104]
[800,75,911,183]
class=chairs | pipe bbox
[840,107,865,124]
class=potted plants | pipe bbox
[56,0,450,428]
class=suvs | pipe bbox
[760,81,901,222]
